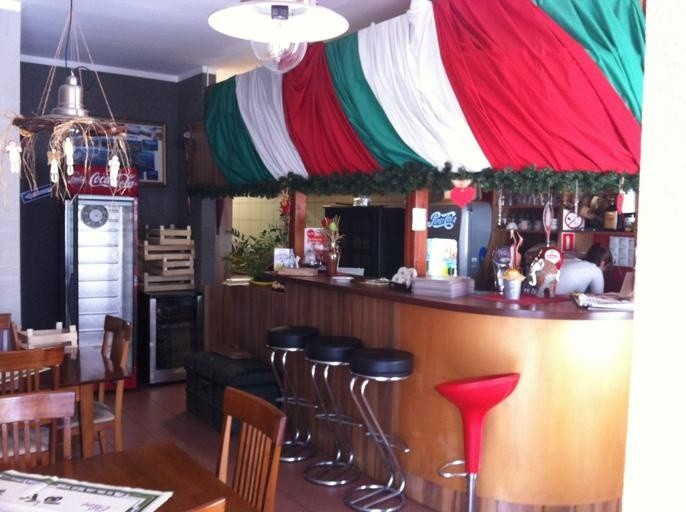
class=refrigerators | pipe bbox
[426,201,493,284]
[22,163,140,394]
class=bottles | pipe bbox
[603,198,619,231]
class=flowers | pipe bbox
[320,214,348,249]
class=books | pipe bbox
[587,305,632,311]
[411,275,477,299]
[571,291,633,308]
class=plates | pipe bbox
[332,276,354,283]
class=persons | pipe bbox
[553,242,613,295]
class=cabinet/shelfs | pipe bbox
[494,204,557,237]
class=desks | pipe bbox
[4,343,133,459]
[0,441,259,512]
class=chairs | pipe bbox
[54,314,131,454]
[216,385,286,510]
[2,312,20,353]
[1,346,63,393]
[0,390,75,471]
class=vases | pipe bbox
[326,249,341,276]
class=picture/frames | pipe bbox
[89,113,168,189]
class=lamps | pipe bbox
[205,0,351,75]
[0,2,137,206]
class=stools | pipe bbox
[434,372,520,507]
[343,350,415,512]
[303,336,365,488]
[266,325,316,463]
[181,351,280,436]
